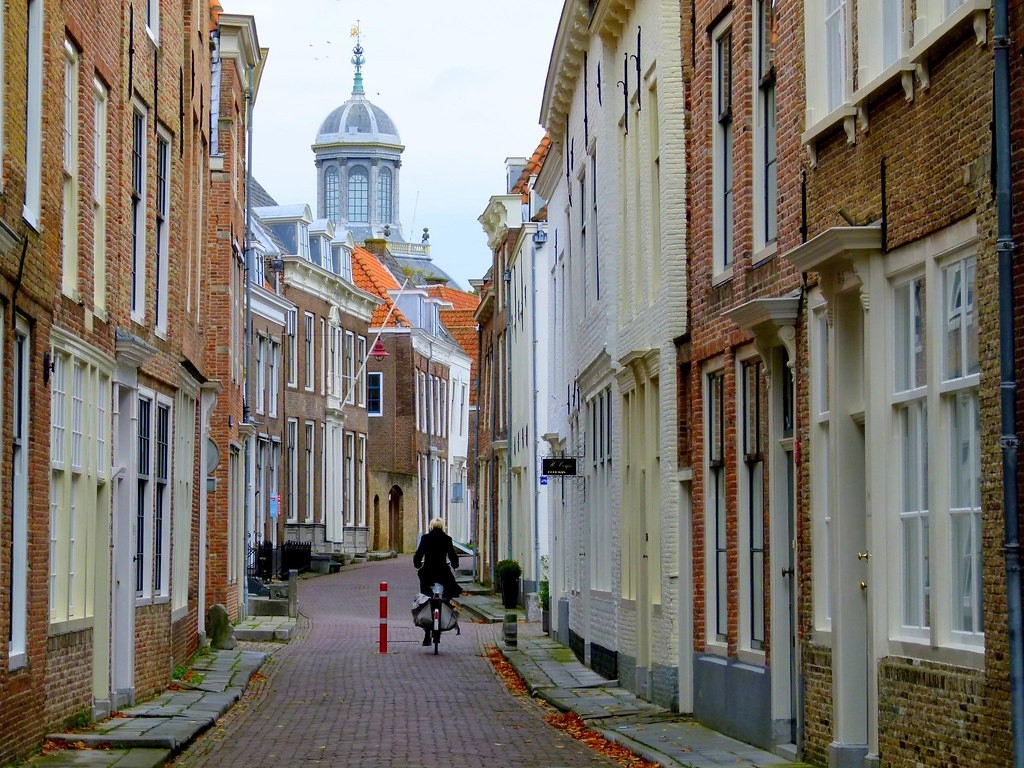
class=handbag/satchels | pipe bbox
[439,598,459,632]
[411,593,434,630]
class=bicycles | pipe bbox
[413,561,458,654]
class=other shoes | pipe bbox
[423,637,431,646]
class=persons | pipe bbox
[412,517,459,647]
